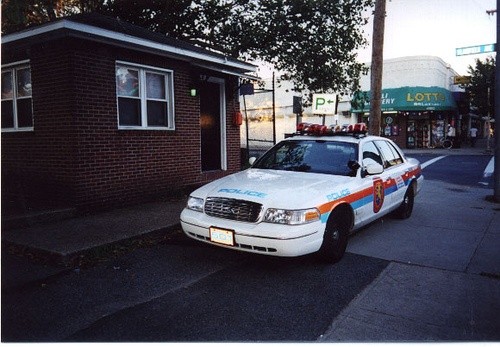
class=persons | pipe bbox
[470,123,479,147]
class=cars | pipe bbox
[178,121,424,265]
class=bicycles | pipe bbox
[425,135,454,149]
